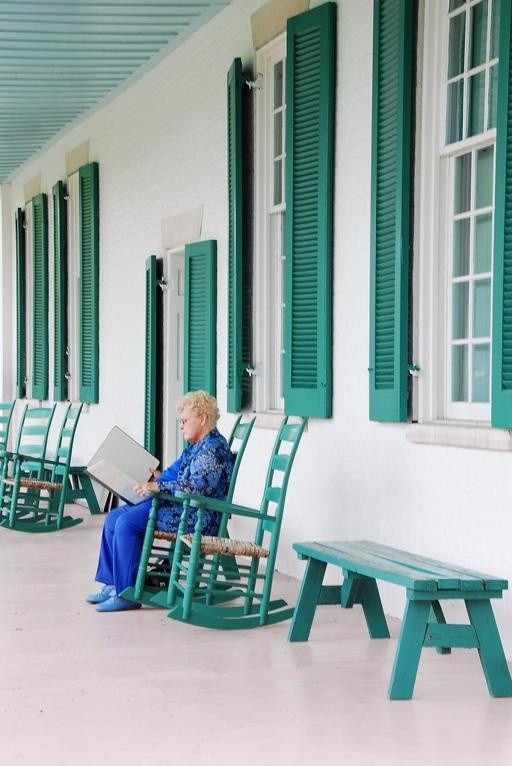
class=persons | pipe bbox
[86,390,234,613]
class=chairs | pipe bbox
[165,417,309,631]
[1,398,103,534]
[115,412,257,608]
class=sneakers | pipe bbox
[86,584,115,603]
[96,590,142,612]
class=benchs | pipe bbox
[287,539,512,700]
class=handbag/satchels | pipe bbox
[145,559,171,588]
